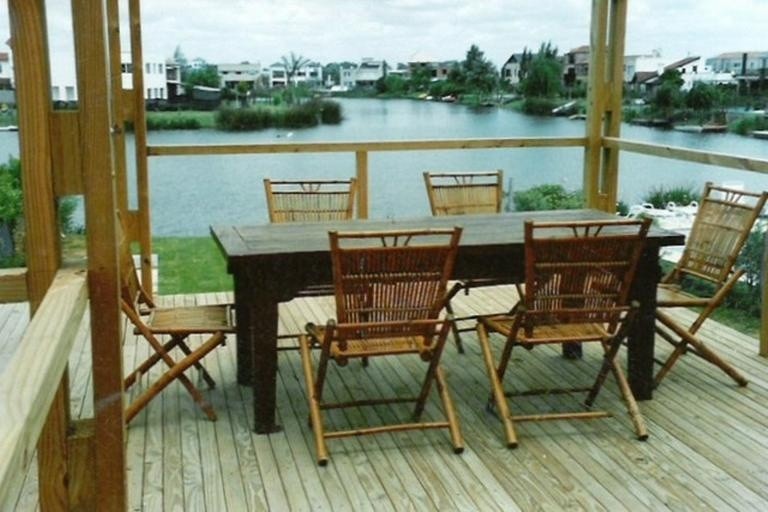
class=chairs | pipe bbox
[104,211,237,429]
[422,168,537,353]
[647,179,767,396]
[260,175,372,375]
[474,215,651,451]
[296,223,467,470]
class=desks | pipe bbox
[208,208,687,436]
